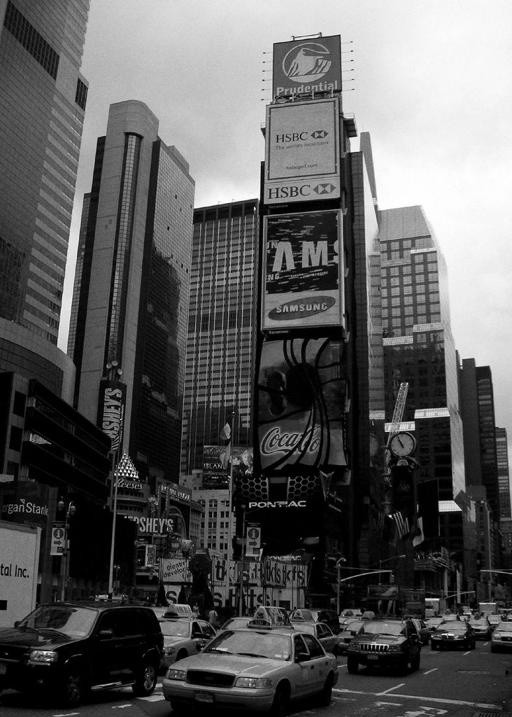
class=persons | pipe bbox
[258,370,289,424]
[209,608,219,626]
[193,602,201,617]
[259,363,347,467]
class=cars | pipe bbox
[411,612,512,652]
[0,600,165,708]
[346,618,421,675]
[154,603,375,717]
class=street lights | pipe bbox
[327,557,347,616]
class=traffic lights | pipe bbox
[390,573,395,584]
